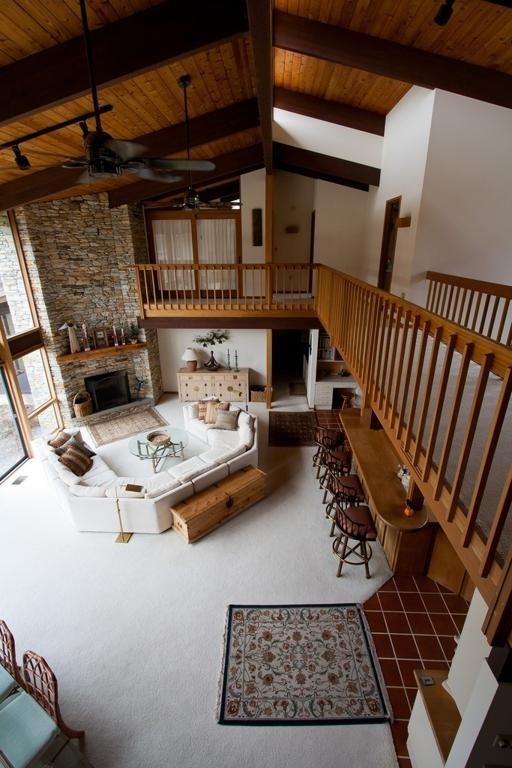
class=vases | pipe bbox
[204,350,221,371]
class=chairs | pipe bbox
[0,619,27,705]
[313,430,345,481]
[1,650,85,768]
[331,499,377,579]
[325,467,365,522]
[312,452,350,504]
[311,425,343,468]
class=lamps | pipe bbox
[11,145,32,172]
[434,1,456,27]
[180,347,201,371]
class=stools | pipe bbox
[340,391,354,409]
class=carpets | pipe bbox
[86,408,169,447]
[267,409,320,447]
[216,602,391,723]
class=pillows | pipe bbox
[48,431,71,447]
[51,430,97,459]
[199,398,219,423]
[208,407,242,431]
[57,443,92,476]
[205,401,230,424]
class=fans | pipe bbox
[2,79,216,183]
[151,76,233,211]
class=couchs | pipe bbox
[47,396,259,535]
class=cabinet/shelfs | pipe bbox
[177,367,250,405]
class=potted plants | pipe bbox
[128,319,139,345]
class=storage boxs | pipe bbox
[170,463,268,544]
